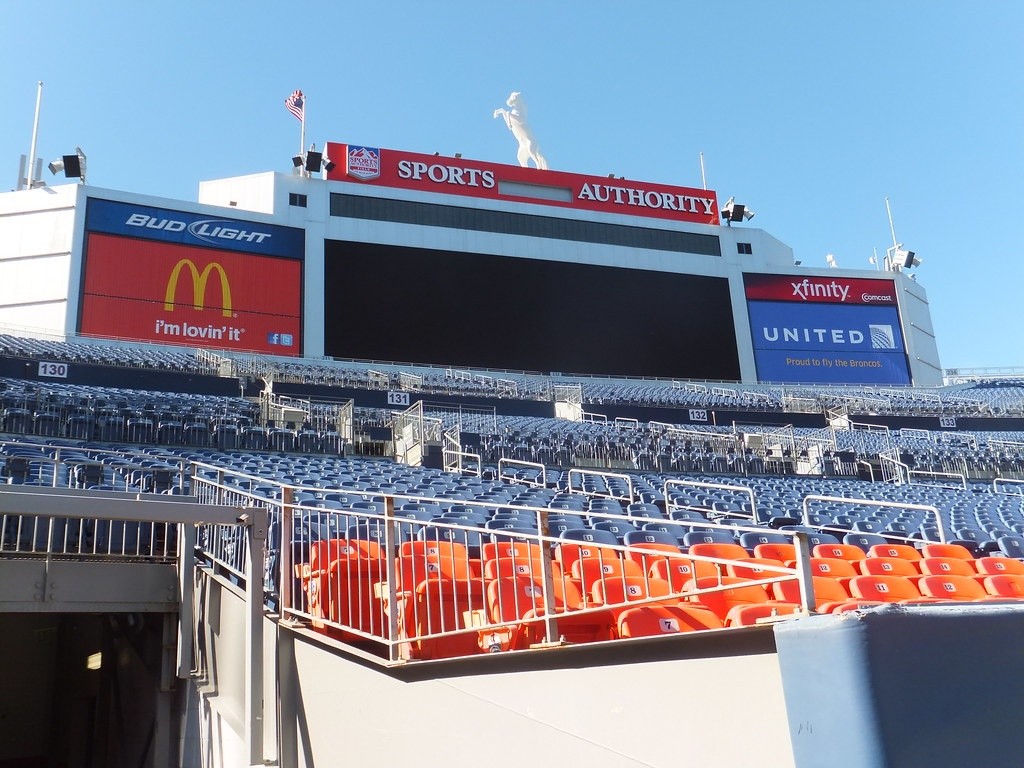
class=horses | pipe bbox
[493,91,550,170]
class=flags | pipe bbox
[285,90,304,121]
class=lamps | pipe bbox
[454,153,462,158]
[291,142,336,179]
[229,201,237,208]
[795,261,801,267]
[720,196,755,227]
[887,243,923,273]
[48,146,86,186]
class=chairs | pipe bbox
[0,335,1024,660]
